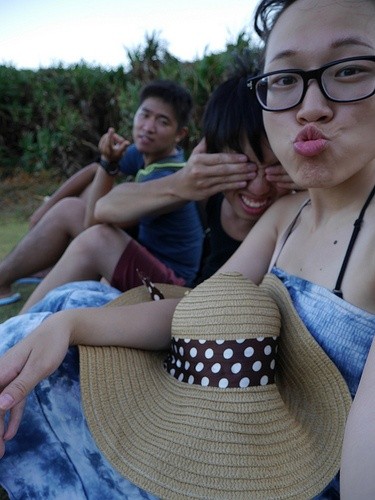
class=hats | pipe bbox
[76,271,353,500]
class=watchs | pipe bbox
[98,159,121,176]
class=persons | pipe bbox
[0,0,375,499]
[15,73,306,314]
[0,81,205,299]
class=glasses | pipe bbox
[248,55,374,112]
